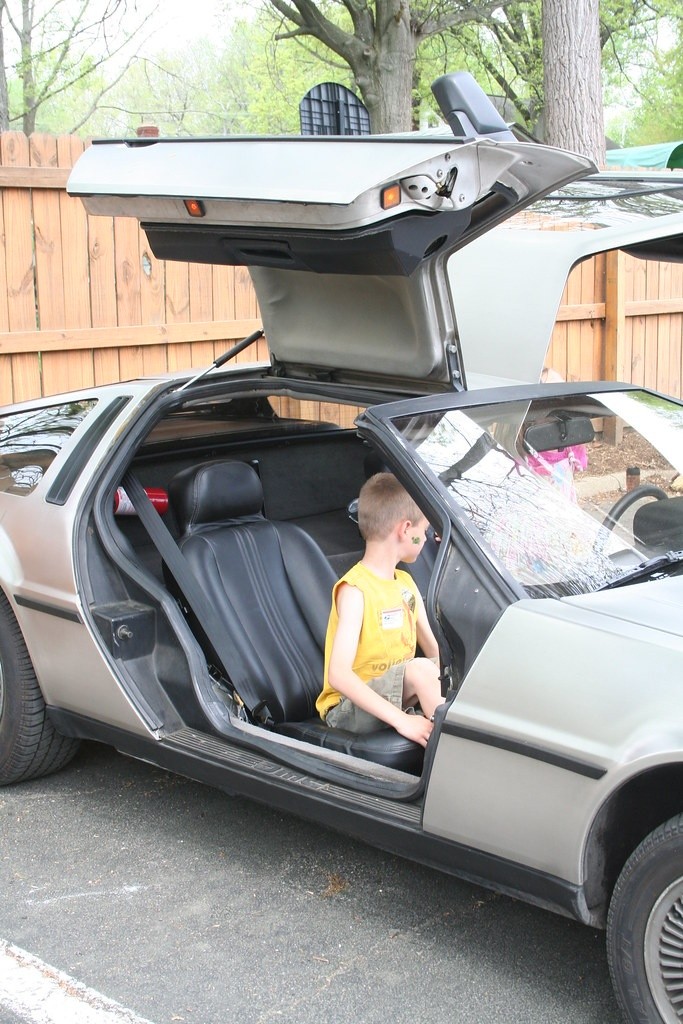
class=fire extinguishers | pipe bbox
[114,489,169,514]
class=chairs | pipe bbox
[162,458,424,775]
[348,439,441,601]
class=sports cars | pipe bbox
[0,69,682,1024]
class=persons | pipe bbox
[515,369,587,505]
[315,472,447,750]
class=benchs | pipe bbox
[119,434,363,601]
